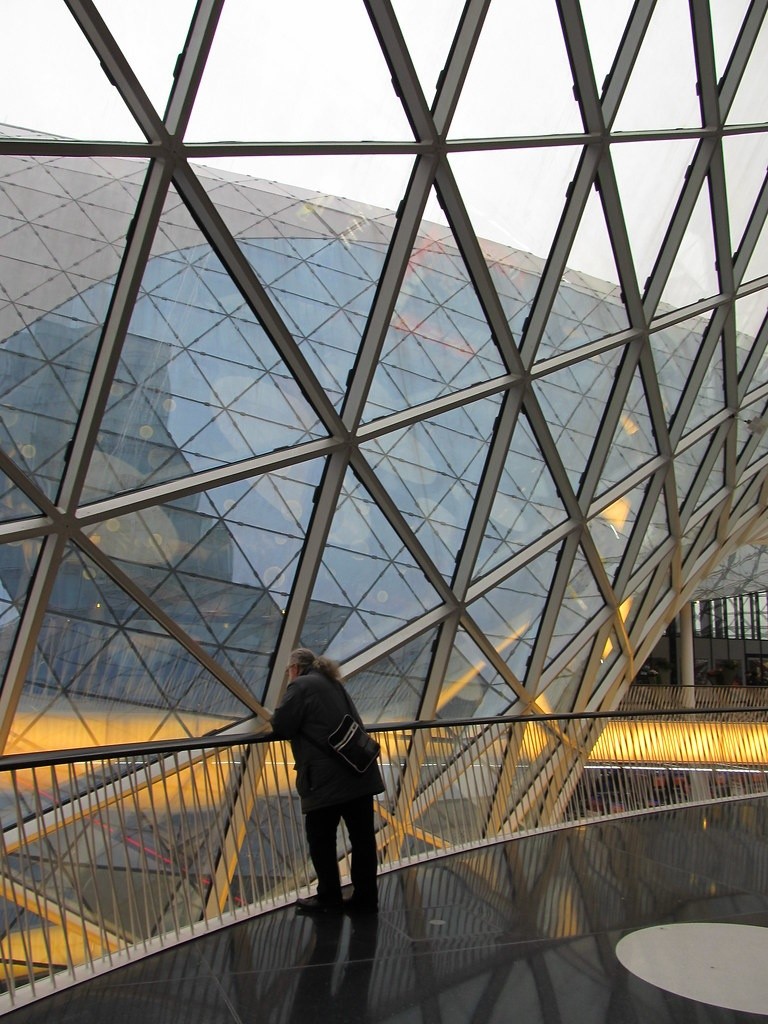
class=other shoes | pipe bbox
[295,896,344,914]
[342,896,378,914]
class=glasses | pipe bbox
[285,664,296,672]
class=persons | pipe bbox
[269,648,386,915]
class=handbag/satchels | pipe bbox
[327,713,380,774]
